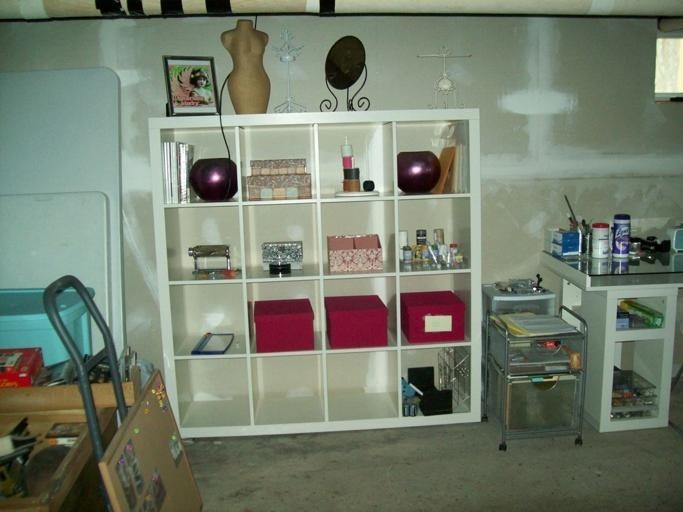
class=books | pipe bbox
[488,310,579,338]
[189,330,235,355]
[431,145,456,195]
[161,141,195,206]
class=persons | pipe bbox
[186,67,215,108]
[219,17,269,116]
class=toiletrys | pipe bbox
[401,228,465,269]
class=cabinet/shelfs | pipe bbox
[146,106,481,439]
[482,278,586,451]
[542,249,683,433]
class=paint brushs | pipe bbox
[564,196,587,224]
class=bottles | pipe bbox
[583,214,659,258]
[403,399,417,417]
[583,255,656,274]
[400,229,463,265]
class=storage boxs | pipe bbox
[0,285,92,365]
[253,290,466,353]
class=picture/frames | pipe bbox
[162,55,221,117]
